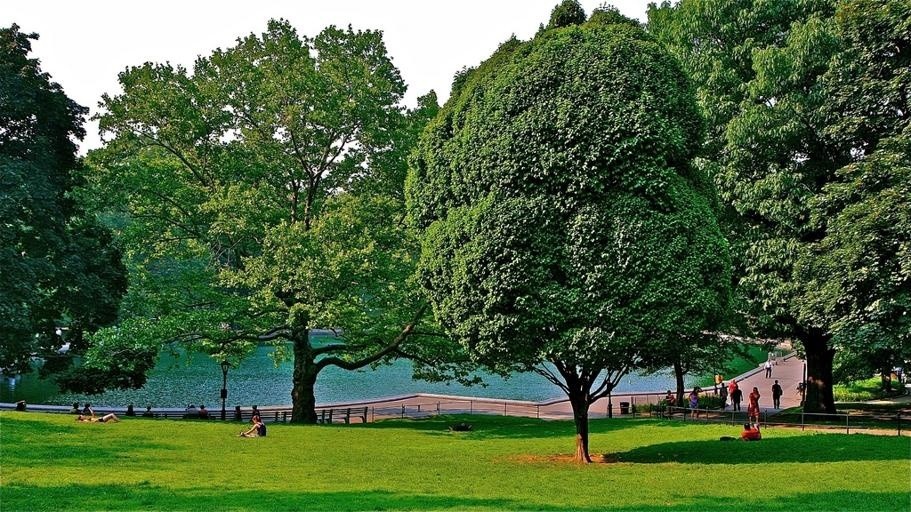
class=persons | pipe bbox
[70,401,119,422]
[188,405,208,420]
[764,358,773,378]
[741,423,761,440]
[688,387,703,422]
[236,405,266,437]
[666,390,675,406]
[126,405,153,417]
[720,379,783,424]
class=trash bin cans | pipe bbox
[620,402,629,414]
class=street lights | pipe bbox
[799,354,808,406]
[221,359,232,420]
[605,357,614,419]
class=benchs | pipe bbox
[46,406,369,424]
